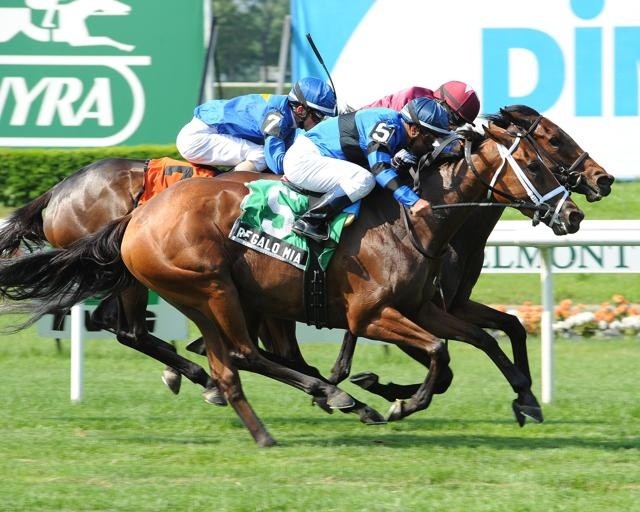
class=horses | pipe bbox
[1,121,584,444]
[216,104,615,415]
[1,158,226,407]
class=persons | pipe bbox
[175,75,339,177]
[358,79,483,164]
[284,94,455,241]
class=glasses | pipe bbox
[413,121,436,145]
[301,104,324,123]
[441,101,465,127]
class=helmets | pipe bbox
[400,96,450,135]
[431,81,480,127]
[287,76,336,118]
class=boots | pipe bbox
[292,185,354,248]
